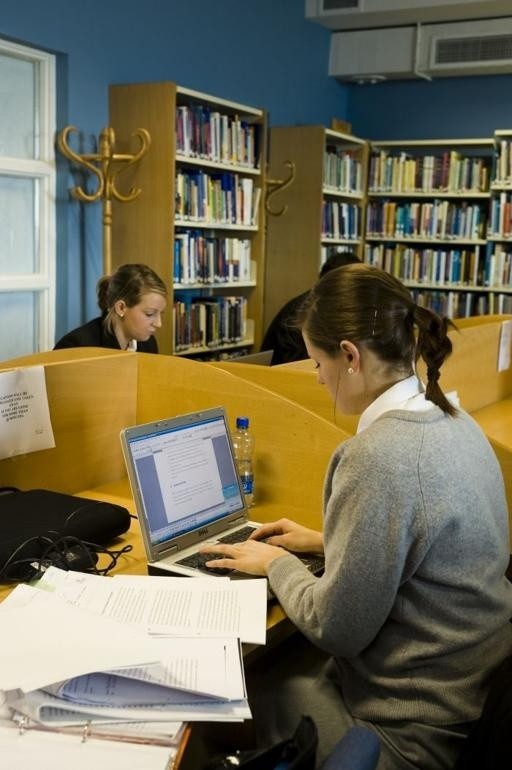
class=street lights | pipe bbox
[0,483,130,584]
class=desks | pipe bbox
[0,316,512,769]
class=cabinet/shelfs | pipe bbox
[107,79,266,363]
[265,123,370,332]
[229,416,254,504]
[366,130,510,314]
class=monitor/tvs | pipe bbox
[319,138,512,320]
[170,95,257,360]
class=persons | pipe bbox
[259,253,365,366]
[48,264,167,353]
[200,262,512,770]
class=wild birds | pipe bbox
[227,349,274,366]
[120,405,325,602]
